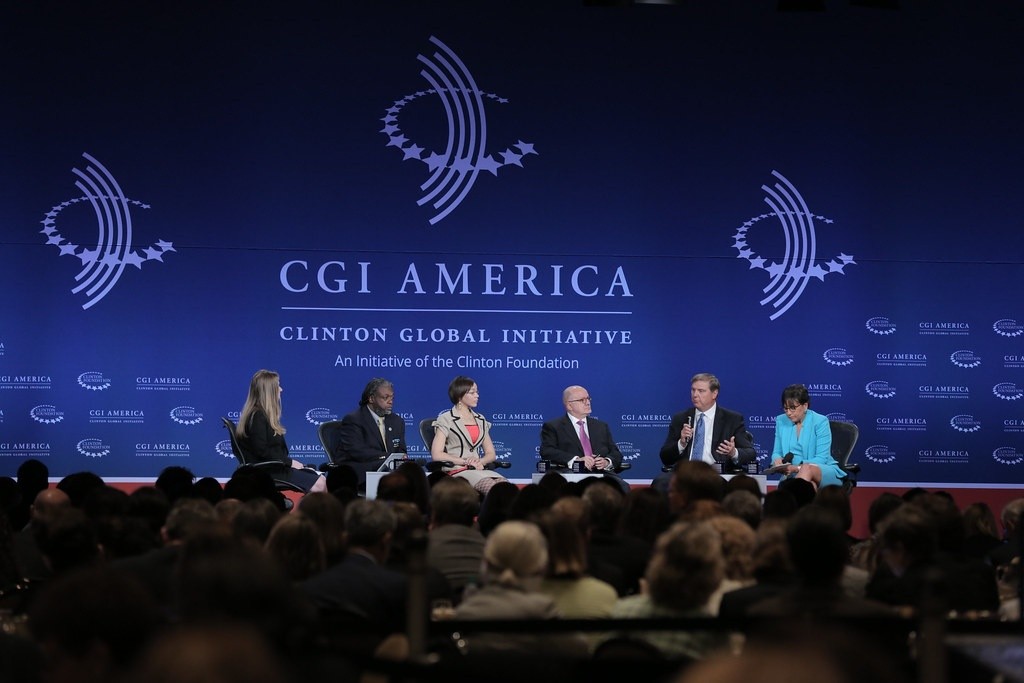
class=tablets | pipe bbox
[376,452,405,472]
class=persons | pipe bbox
[1,460,1024,683]
[538,385,631,495]
[335,373,408,478]
[658,372,755,474]
[769,384,849,494]
[431,374,507,495]
[233,368,326,495]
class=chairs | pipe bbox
[415,417,512,475]
[218,417,318,472]
[827,422,863,491]
[316,421,349,467]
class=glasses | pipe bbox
[567,396,592,403]
[464,390,479,395]
[782,403,802,411]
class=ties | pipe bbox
[378,416,389,453]
[576,421,593,457]
[691,413,705,461]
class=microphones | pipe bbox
[467,463,495,470]
[686,408,695,442]
[303,464,317,470]
[768,452,794,468]
